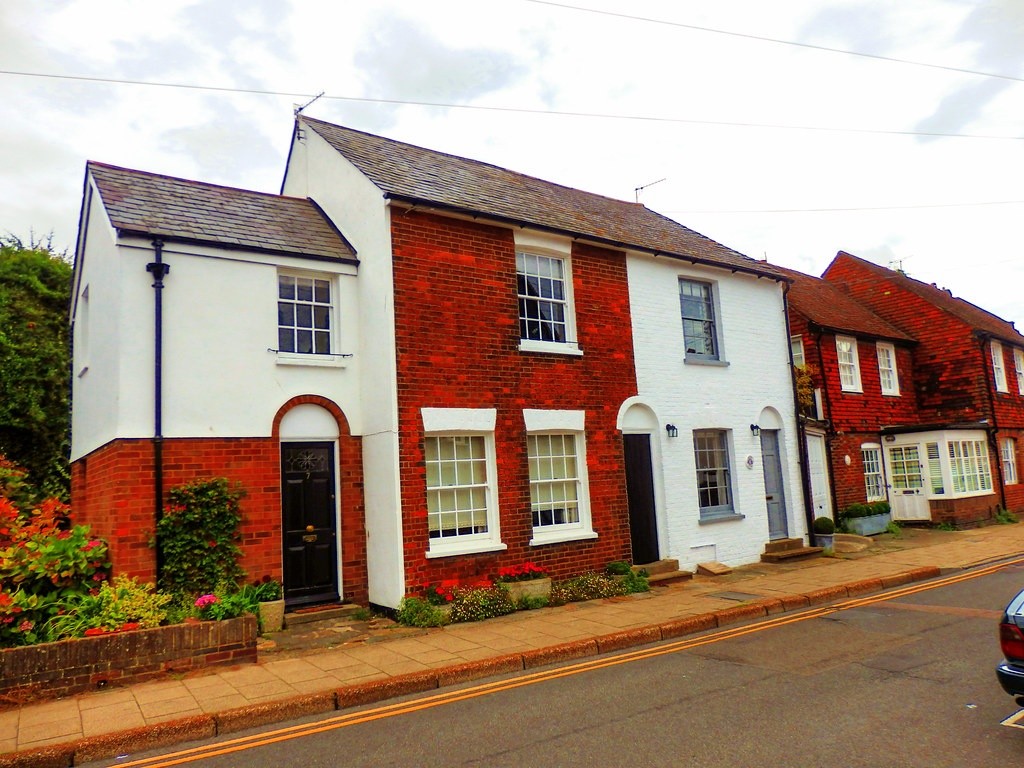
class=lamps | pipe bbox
[666,423,678,437]
[750,424,761,436]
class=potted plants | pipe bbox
[85,598,137,637]
[607,560,631,586]
[843,502,891,536]
[813,516,835,551]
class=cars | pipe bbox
[995,589,1024,708]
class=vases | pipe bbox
[434,603,452,614]
[499,578,552,603]
[256,601,286,634]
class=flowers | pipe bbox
[253,574,285,601]
[498,562,550,583]
[422,579,459,606]
